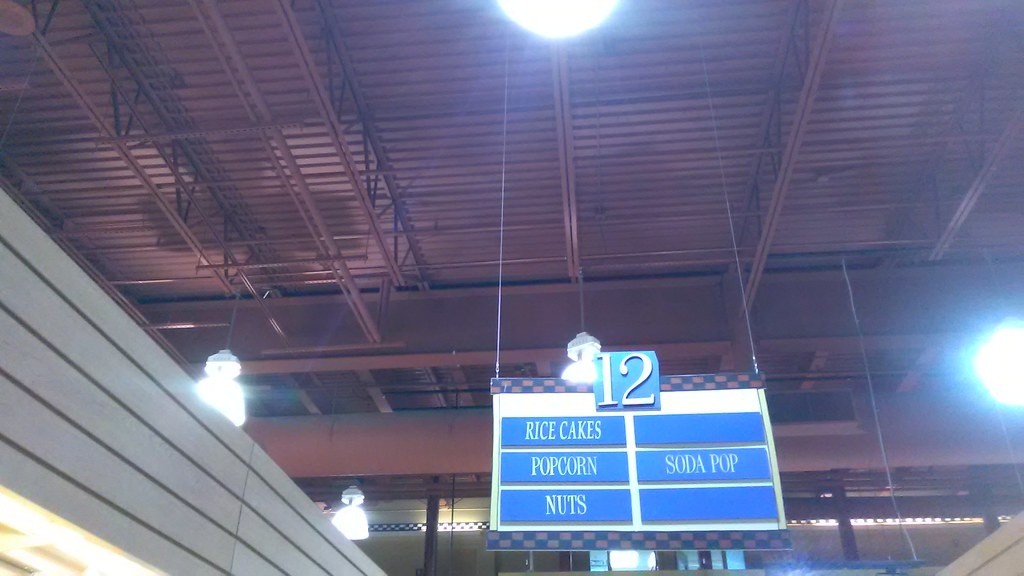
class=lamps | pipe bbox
[561,265,601,382]
[196,280,246,428]
[332,481,369,541]
